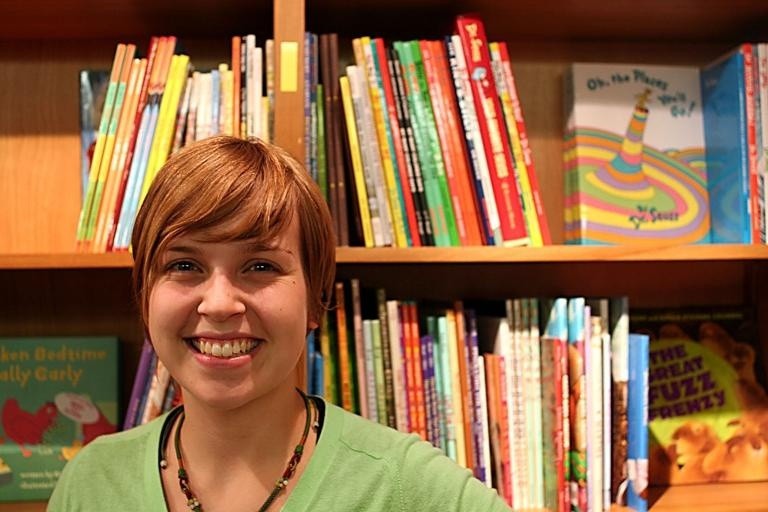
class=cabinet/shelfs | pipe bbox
[0,0,767,512]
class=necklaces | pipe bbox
[160,387,311,512]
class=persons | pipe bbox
[45,135,516,512]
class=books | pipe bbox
[304,17,555,247]
[80,35,272,253]
[629,305,768,486]
[121,336,184,440]
[306,278,651,512]
[562,43,765,245]
[0,335,121,504]
